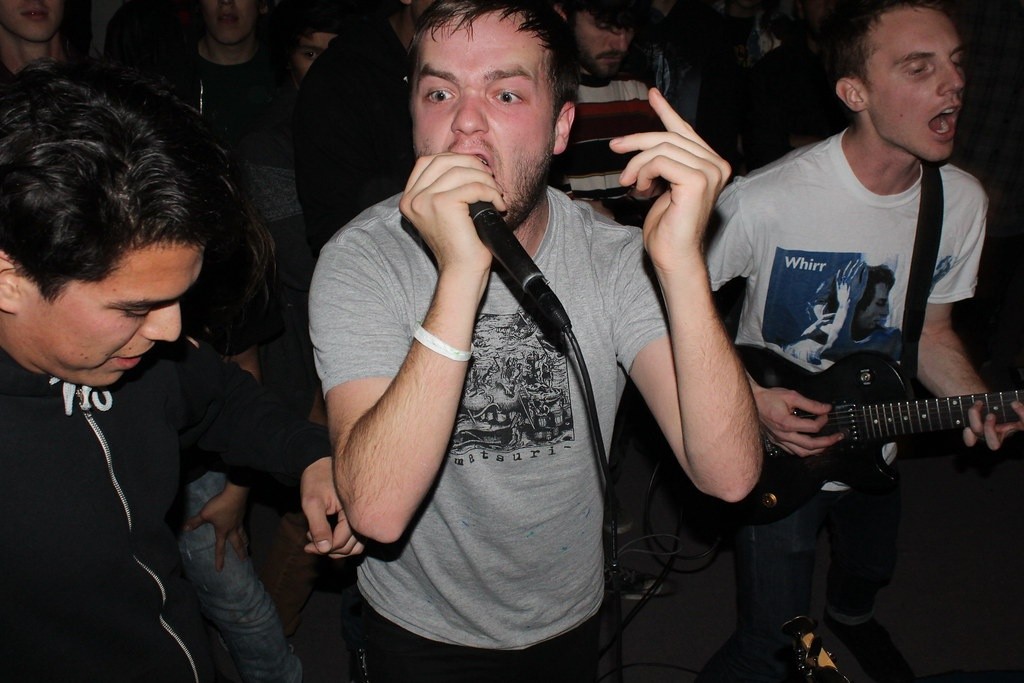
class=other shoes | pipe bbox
[823,608,916,683]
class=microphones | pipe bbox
[467,201,571,332]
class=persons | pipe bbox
[707,1,1024,683]
[309,0,762,683]
[0,58,363,683]
[0,0,1024,640]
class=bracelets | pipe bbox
[414,321,473,361]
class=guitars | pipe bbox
[693,337,1024,524]
